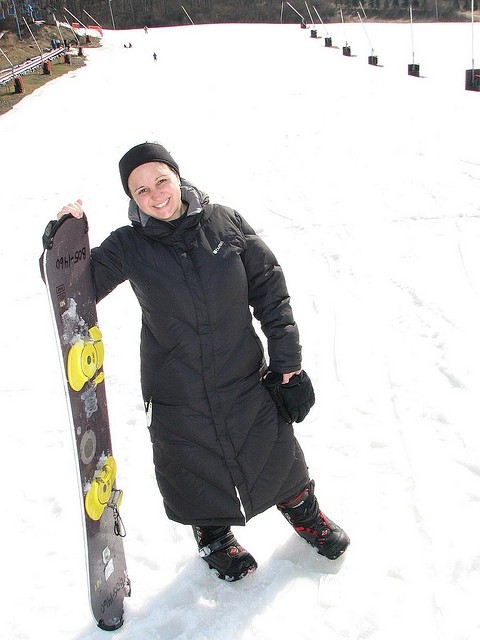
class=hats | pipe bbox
[119,142,180,201]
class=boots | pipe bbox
[193,524,258,582]
[276,479,350,561]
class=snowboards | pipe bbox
[43,210,131,630]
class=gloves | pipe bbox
[266,372,315,425]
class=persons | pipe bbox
[38,141,349,582]
